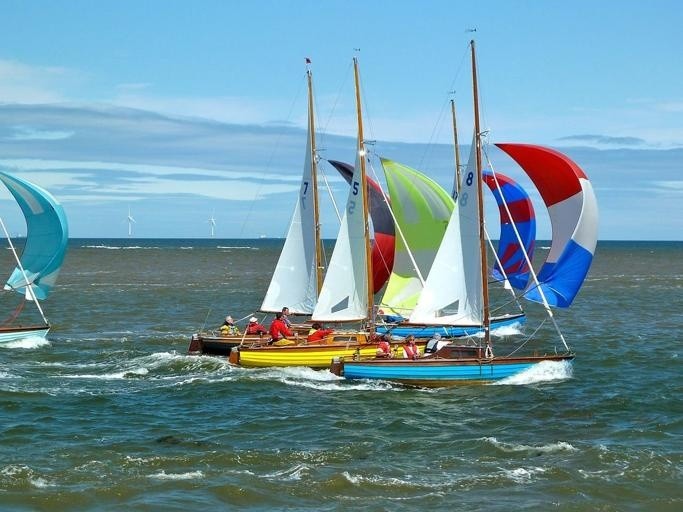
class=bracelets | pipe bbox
[393,348,397,352]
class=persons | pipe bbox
[423,333,454,357]
[281,307,291,328]
[220,316,241,336]
[308,323,335,342]
[270,312,297,340]
[376,334,399,359]
[401,335,421,358]
[246,317,270,335]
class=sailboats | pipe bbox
[232,48,450,369]
[341,28,579,388]
[187,53,364,351]
[0,169,73,351]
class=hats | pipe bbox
[250,317,258,323]
